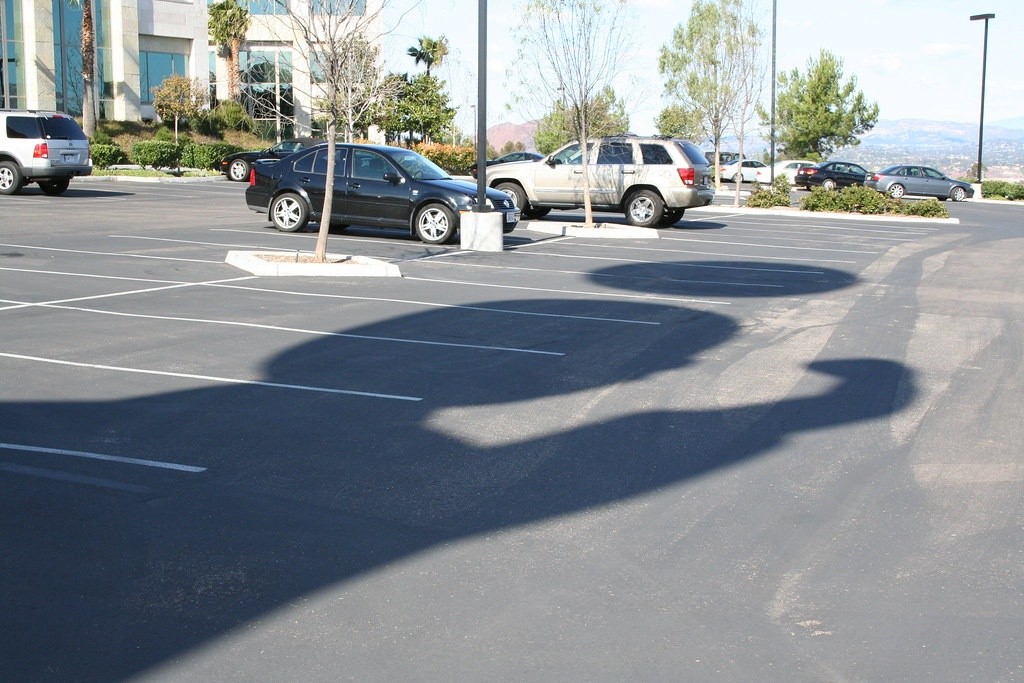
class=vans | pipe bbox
[704,151,747,167]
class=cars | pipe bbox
[718,159,771,184]
[219,137,342,182]
[470,152,545,181]
[864,163,974,202]
[793,161,868,193]
[755,159,818,187]
[245,143,521,246]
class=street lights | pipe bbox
[969,12,995,183]
[470,105,475,161]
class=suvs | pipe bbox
[485,133,716,229]
[0,108,93,196]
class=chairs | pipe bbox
[837,167,841,171]
[368,158,385,178]
[908,171,919,176]
